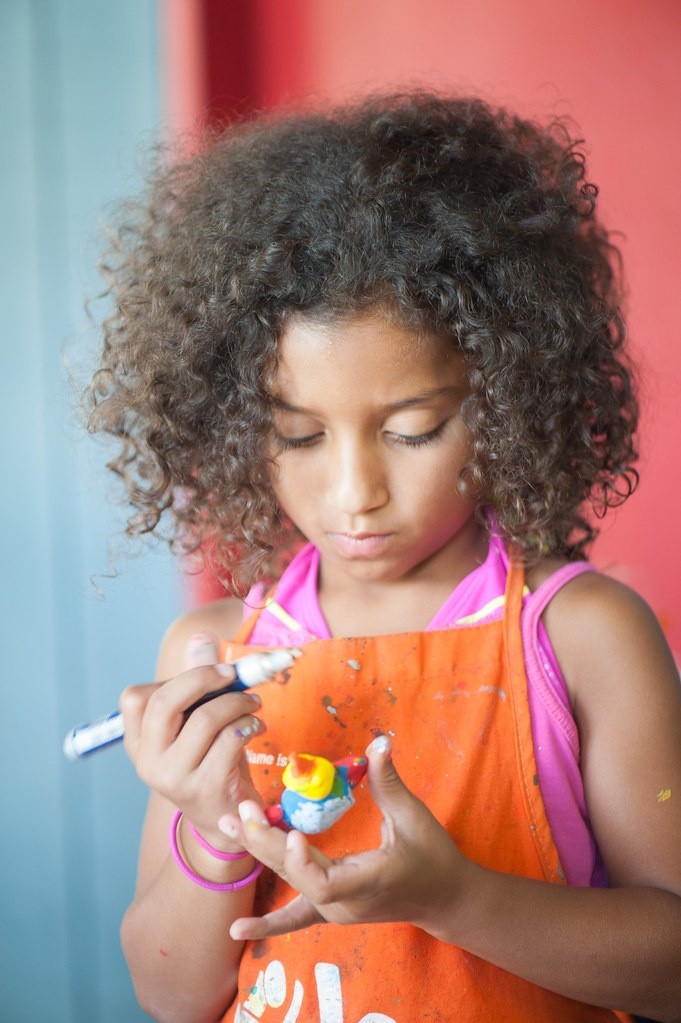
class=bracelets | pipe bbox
[172,810,266,891]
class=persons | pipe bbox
[79,84,681,1023]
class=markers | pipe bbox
[60,645,304,764]
